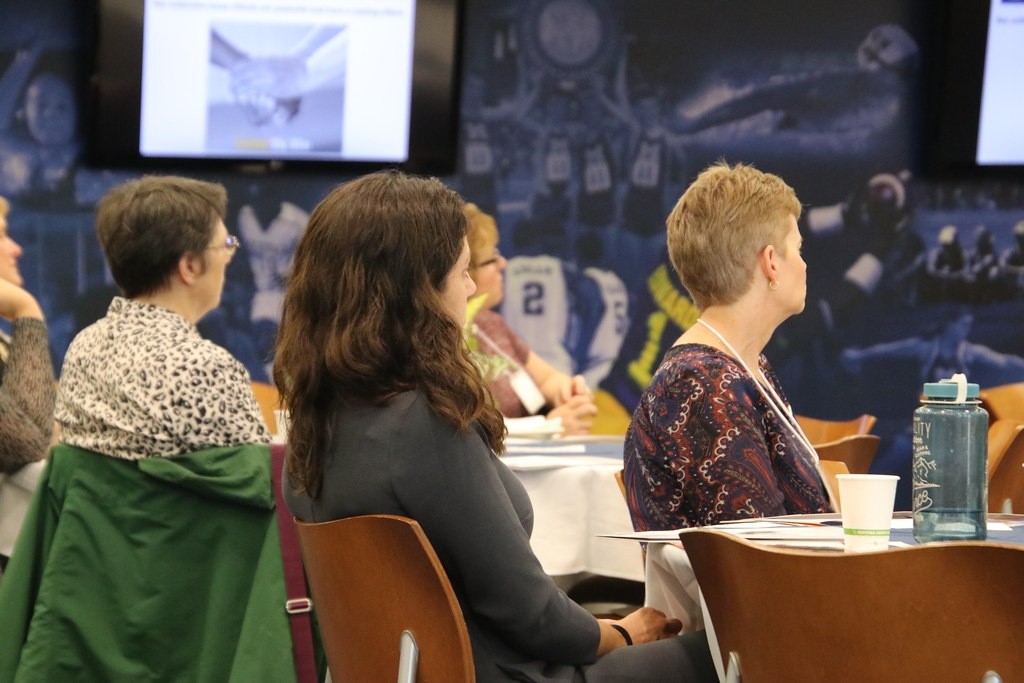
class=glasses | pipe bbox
[204,234,240,256]
[470,249,503,271]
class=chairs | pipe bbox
[252,381,1023,683]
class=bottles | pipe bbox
[912,373,986,542]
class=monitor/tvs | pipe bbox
[78,1,468,180]
[960,1,1023,184]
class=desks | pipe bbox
[494,436,647,587]
[638,512,1023,683]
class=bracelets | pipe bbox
[611,623,635,646]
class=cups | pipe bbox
[836,475,900,549]
[275,410,291,440]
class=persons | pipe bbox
[0,26,1023,472]
[613,154,838,530]
[53,174,269,462]
[1,194,55,467]
[268,172,719,683]
[449,201,599,438]
